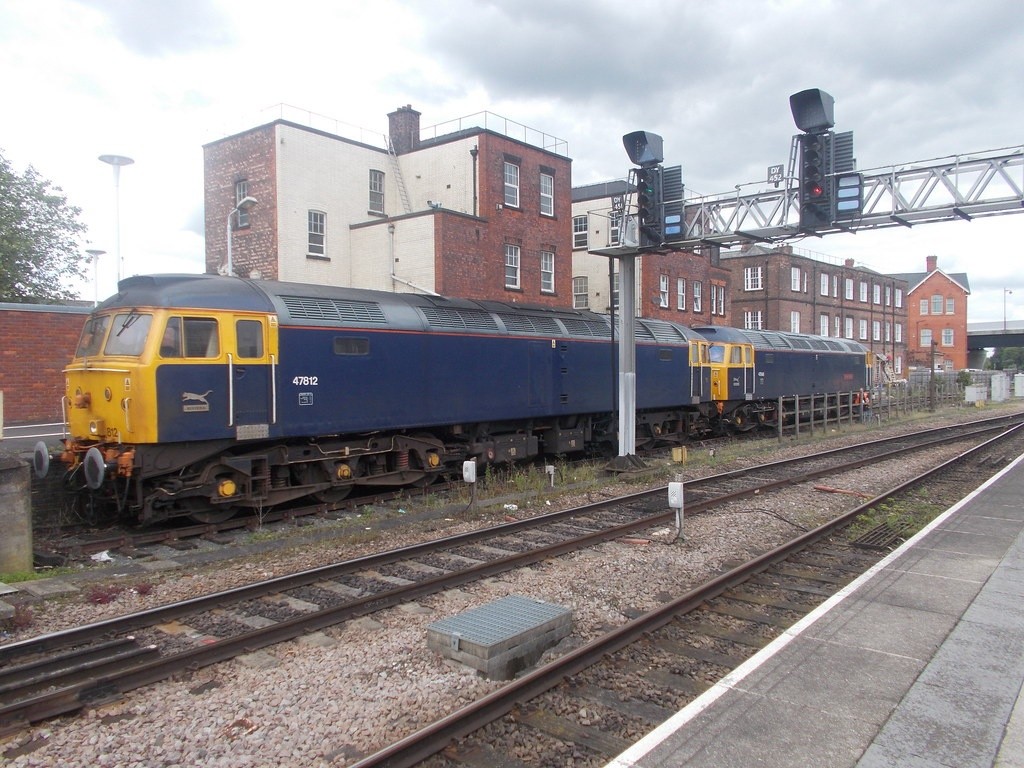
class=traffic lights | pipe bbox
[802,135,828,202]
[639,168,660,224]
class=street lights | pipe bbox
[227,196,259,277]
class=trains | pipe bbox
[33,274,869,530]
